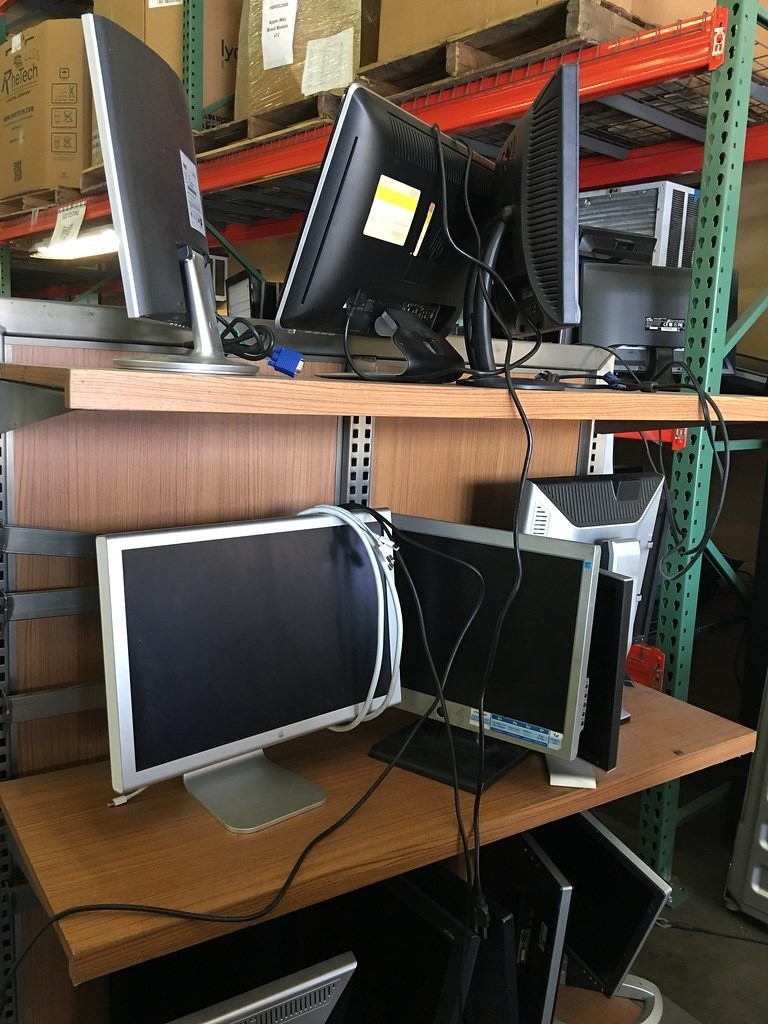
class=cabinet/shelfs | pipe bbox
[0,373,768,1024]
[0,0,768,907]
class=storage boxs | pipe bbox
[90,1,241,164]
[378,0,556,63]
[0,18,91,201]
[235,0,372,120]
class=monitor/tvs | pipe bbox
[97,508,402,833]
[81,13,738,389]
[367,471,668,793]
[165,807,672,1024]
